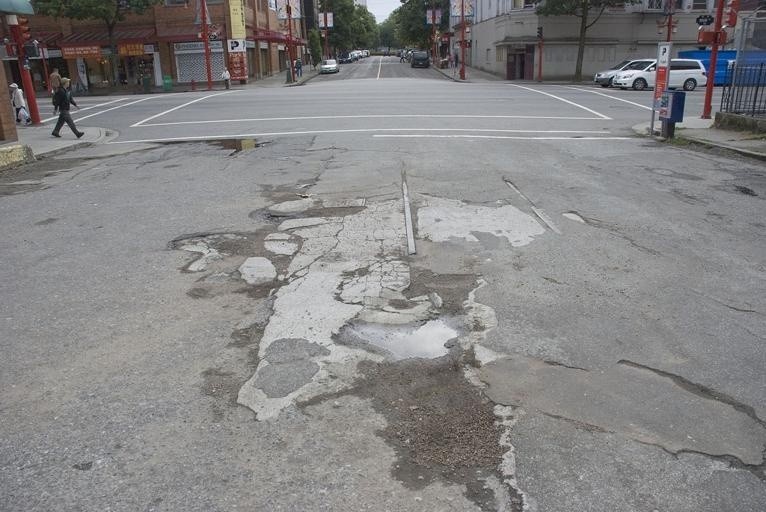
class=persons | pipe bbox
[221,66,231,90]
[296,58,302,77]
[117,65,127,89]
[52,77,84,139]
[34,69,42,92]
[450,54,458,68]
[400,49,405,63]
[49,67,62,112]
[9,83,32,125]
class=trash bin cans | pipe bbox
[163,76,172,91]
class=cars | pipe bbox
[340,49,370,63]
[320,59,340,74]
[594,61,631,88]
[401,49,430,68]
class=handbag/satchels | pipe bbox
[52,92,60,106]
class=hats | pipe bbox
[9,83,18,88]
[60,77,70,85]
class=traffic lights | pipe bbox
[537,27,542,37]
[19,18,32,41]
[726,0,739,26]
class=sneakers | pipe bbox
[77,132,84,138]
[52,133,61,137]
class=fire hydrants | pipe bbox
[191,80,195,90]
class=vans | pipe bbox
[614,58,709,90]
